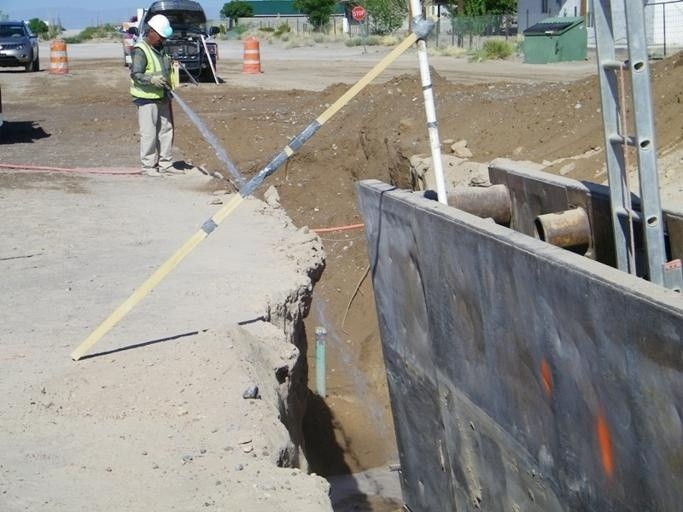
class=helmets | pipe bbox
[148,14,173,39]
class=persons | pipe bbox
[128,14,182,179]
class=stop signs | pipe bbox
[352,6,365,21]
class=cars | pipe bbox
[120,0,217,82]
[0,19,39,72]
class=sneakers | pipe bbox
[141,166,184,177]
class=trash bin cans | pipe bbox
[523,16,587,64]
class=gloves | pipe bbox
[151,74,168,89]
